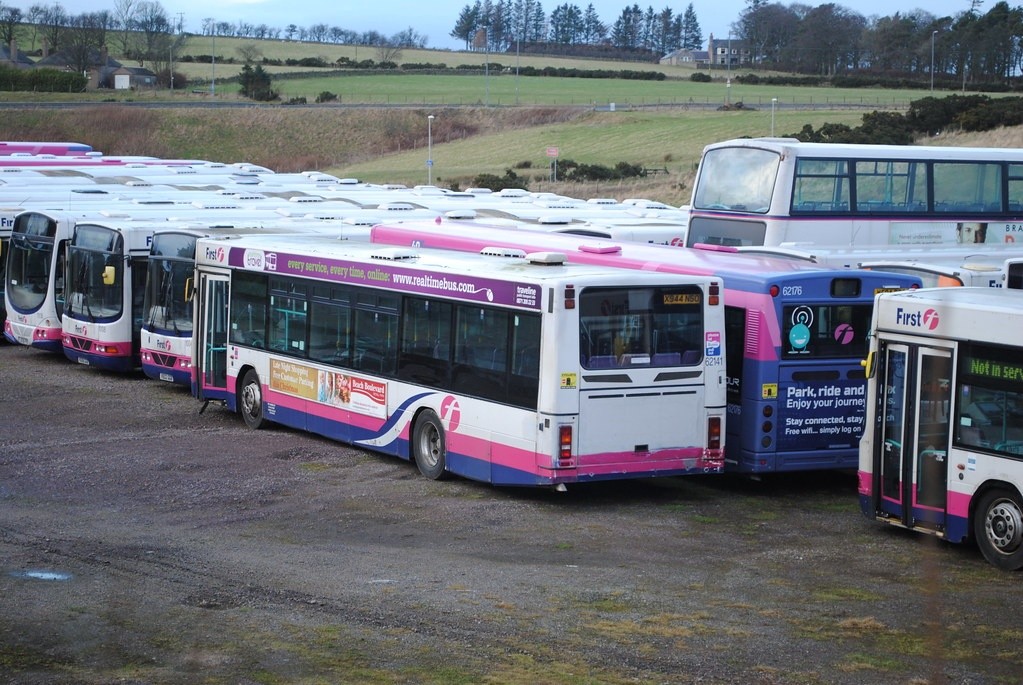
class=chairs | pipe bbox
[803,198,1022,213]
[251,333,540,380]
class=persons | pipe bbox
[955,223,988,244]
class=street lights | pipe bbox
[427,114,436,184]
[516,28,524,104]
[169,46,173,89]
[212,34,215,93]
[771,98,777,137]
[727,29,734,104]
[930,30,938,96]
[483,26,488,108]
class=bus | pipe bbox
[186,229,728,491]
[858,286,1023,568]
[683,138,1023,252]
[1,140,690,385]
[374,214,921,481]
